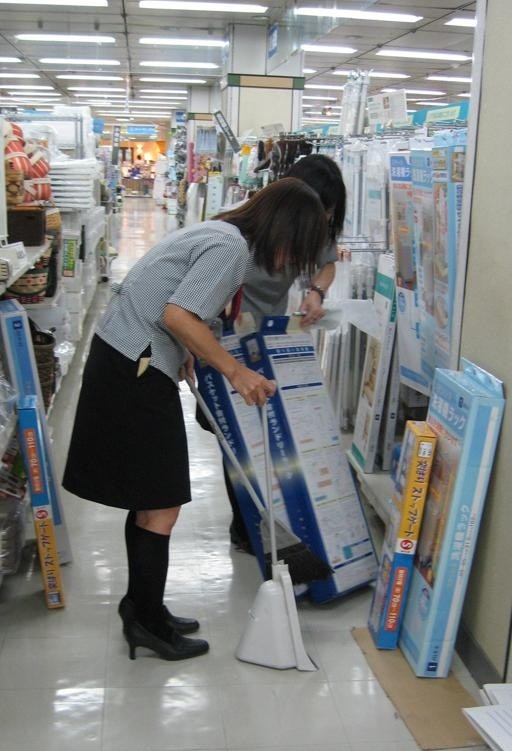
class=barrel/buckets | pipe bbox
[32,329,57,414]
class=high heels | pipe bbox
[117,593,211,661]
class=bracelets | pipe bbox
[308,284,325,304]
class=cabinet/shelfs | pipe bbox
[0,125,77,582]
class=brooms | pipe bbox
[177,367,335,584]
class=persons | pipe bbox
[134,155,145,174]
[195,153,347,557]
[61,177,329,662]
